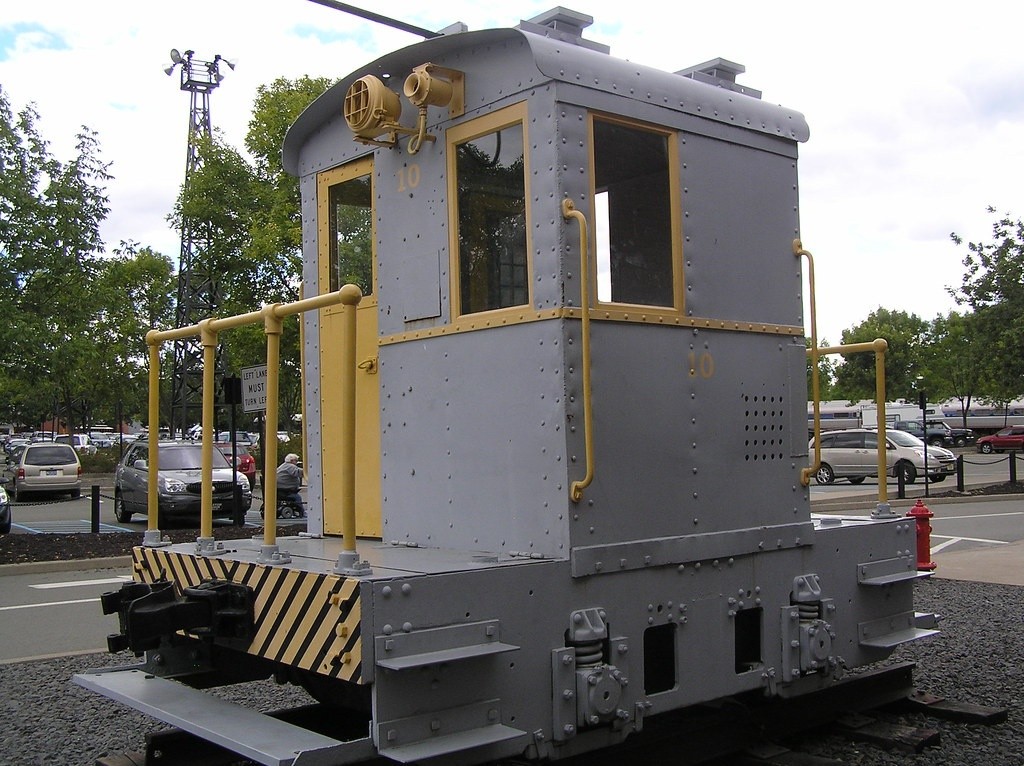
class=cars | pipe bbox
[216,442,257,491]
[3,441,83,498]
[977,424,1024,455]
[1,423,291,456]
[808,429,957,485]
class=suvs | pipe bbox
[919,420,975,448]
[114,433,252,528]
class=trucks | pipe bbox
[859,402,948,430]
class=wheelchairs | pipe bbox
[259,474,307,519]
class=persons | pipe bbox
[277,454,308,518]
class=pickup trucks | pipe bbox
[889,418,950,447]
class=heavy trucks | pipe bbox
[807,396,914,433]
[941,395,1024,416]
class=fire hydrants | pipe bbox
[906,499,937,581]
